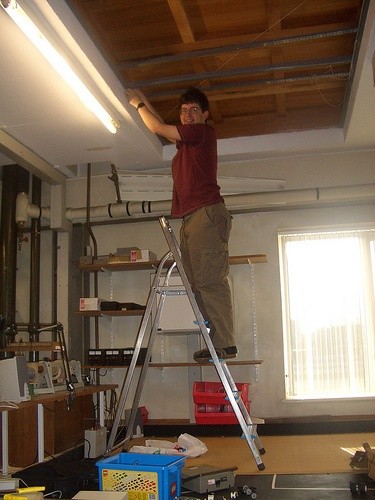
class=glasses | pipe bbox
[178,107,204,115]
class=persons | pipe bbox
[125,86,238,361]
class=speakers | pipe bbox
[0,355,31,403]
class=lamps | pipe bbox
[1,0,120,134]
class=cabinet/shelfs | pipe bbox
[0,384,119,480]
[76,253,269,433]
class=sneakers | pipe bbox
[193,345,237,362]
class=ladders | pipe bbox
[102,217,266,470]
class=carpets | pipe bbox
[106,432,375,475]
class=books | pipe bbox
[0,478,20,492]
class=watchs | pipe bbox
[136,102,145,111]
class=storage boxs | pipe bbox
[193,381,251,404]
[96,452,188,500]
[194,400,253,425]
